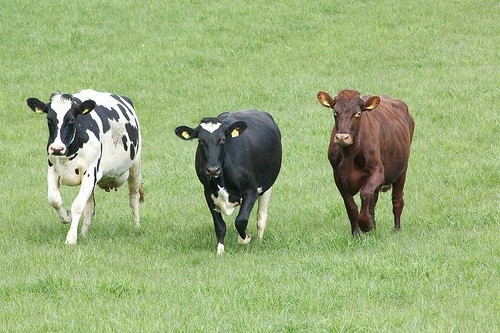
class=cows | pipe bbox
[317,89,414,239]
[175,108,282,256]
[26,88,144,246]
